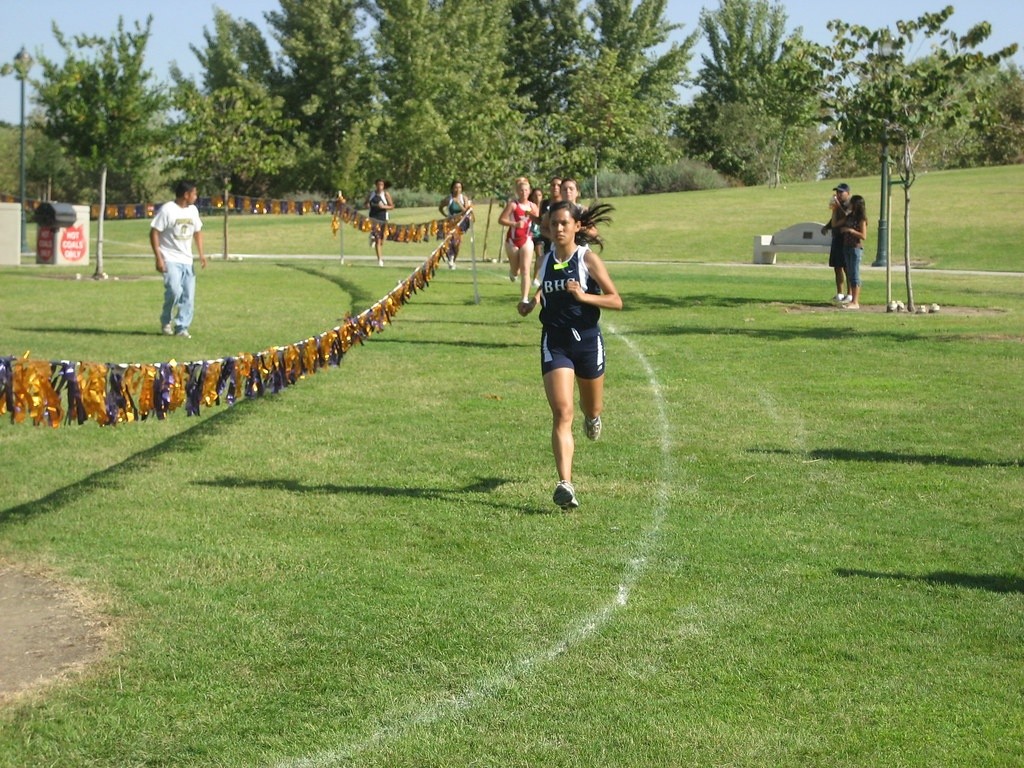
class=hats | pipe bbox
[833,184,850,193]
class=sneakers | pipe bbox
[579,400,602,441]
[552,480,579,514]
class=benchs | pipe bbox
[751,220,832,264]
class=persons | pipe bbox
[517,200,623,510]
[498,177,599,304]
[821,183,867,309]
[150,180,207,339]
[367,178,394,267]
[439,180,469,270]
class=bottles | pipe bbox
[830,194,837,208]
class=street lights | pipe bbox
[875,25,897,265]
[14,44,33,252]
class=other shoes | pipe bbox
[830,294,845,302]
[840,303,860,310]
[174,329,191,339]
[510,270,518,282]
[369,233,376,249]
[448,254,455,271]
[838,296,852,305]
[162,325,173,335]
[377,260,383,267]
[531,278,540,288]
